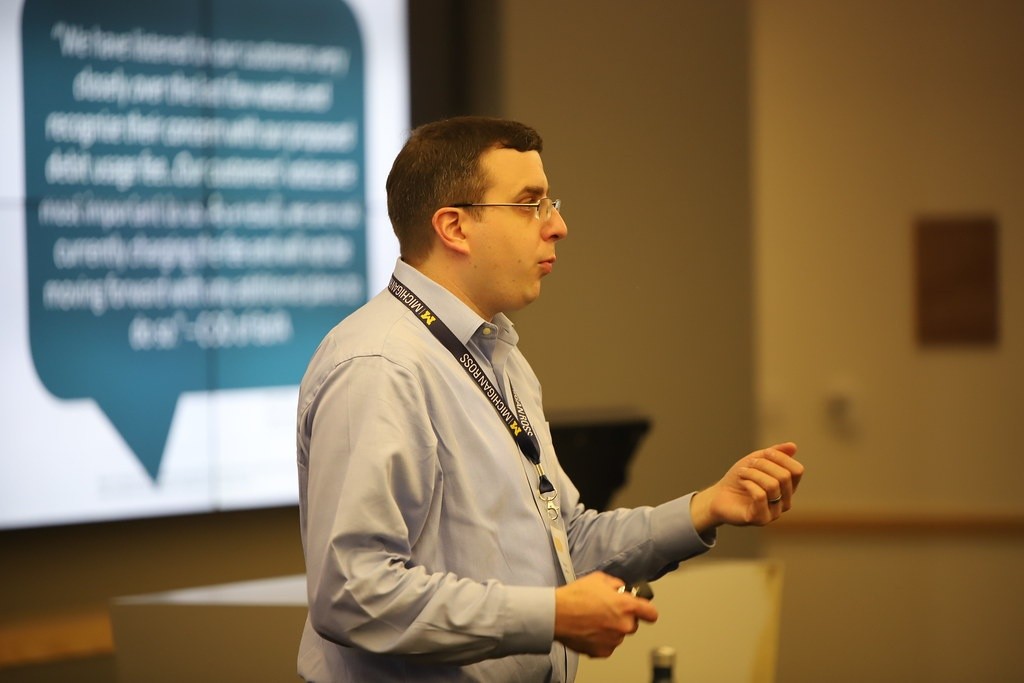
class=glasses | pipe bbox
[447,196,562,221]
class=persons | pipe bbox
[292,112,803,682]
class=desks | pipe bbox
[110,552,781,683]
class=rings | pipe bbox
[768,494,782,504]
